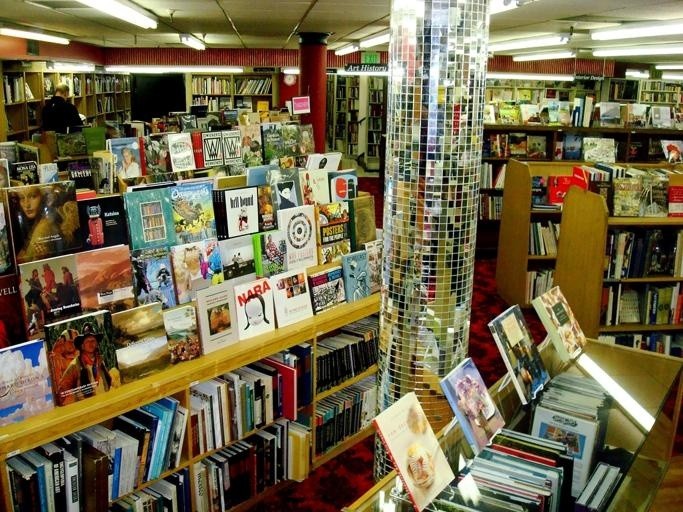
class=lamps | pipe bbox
[487,0,683,69]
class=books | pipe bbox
[0,75,683,512]
